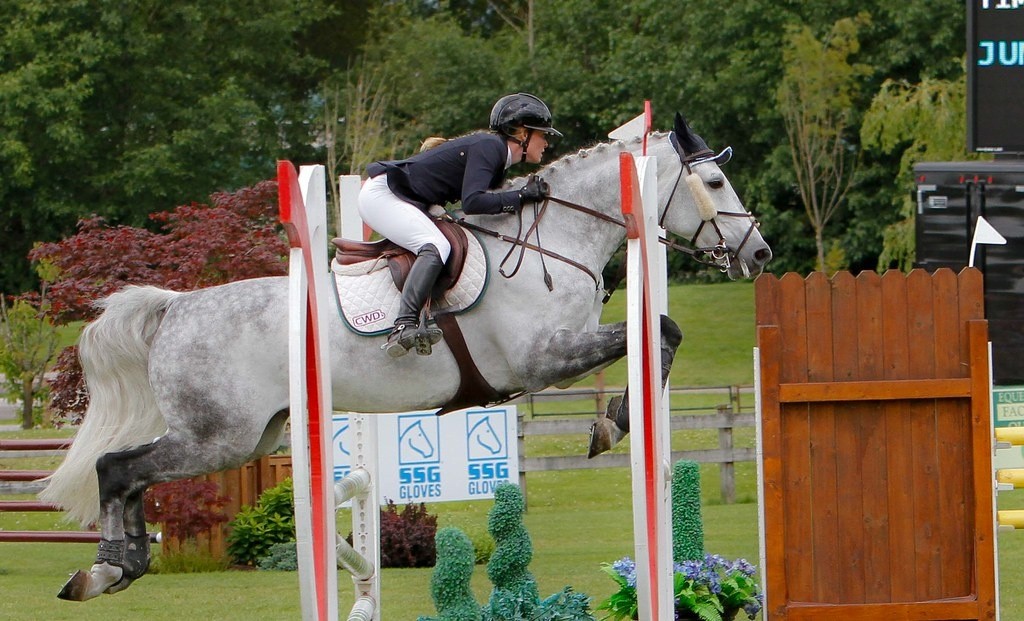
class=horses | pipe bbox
[29,112,773,603]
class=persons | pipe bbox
[357,91,564,357]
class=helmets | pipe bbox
[490,92,564,139]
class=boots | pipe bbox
[385,242,444,358]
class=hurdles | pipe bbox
[275,151,677,621]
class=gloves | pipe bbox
[521,176,547,203]
